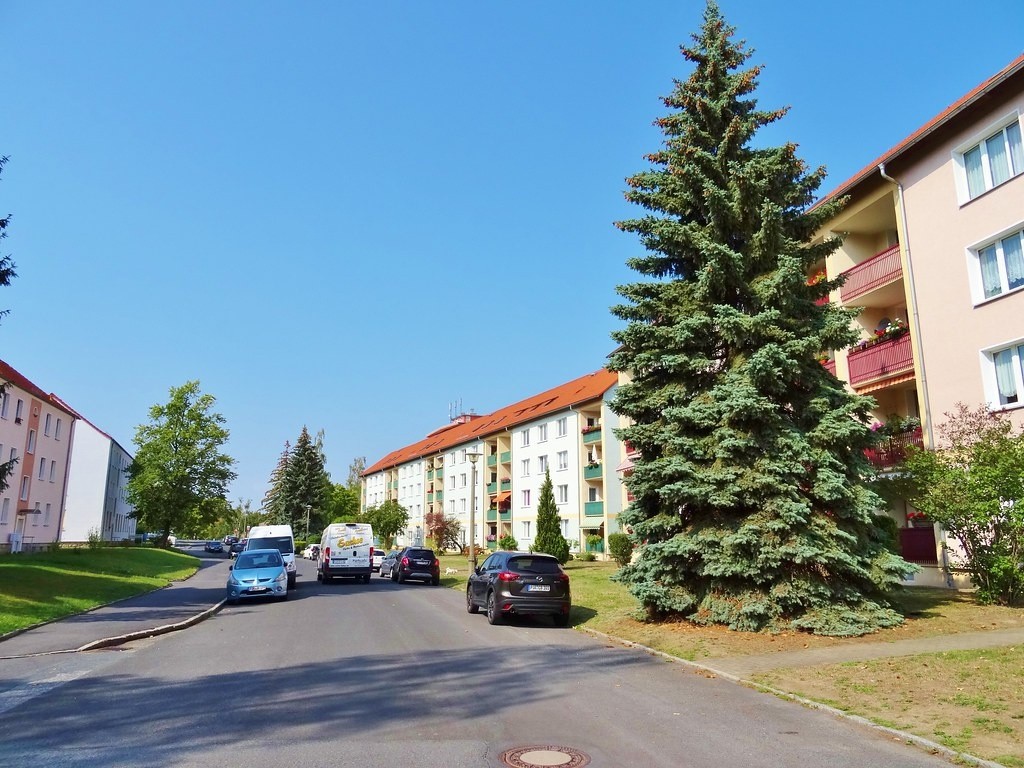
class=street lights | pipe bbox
[306,505,312,546]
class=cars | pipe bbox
[379,550,402,579]
[224,535,239,545]
[302,544,321,560]
[309,545,320,561]
[239,538,248,545]
[467,551,572,628]
[205,541,223,552]
[371,549,387,573]
[227,548,287,603]
[227,543,246,560]
[389,545,440,586]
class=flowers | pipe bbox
[905,512,931,520]
[859,317,908,347]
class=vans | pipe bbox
[245,525,296,589]
[316,522,374,583]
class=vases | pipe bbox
[848,335,890,354]
[912,519,933,528]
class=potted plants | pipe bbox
[816,355,830,365]
